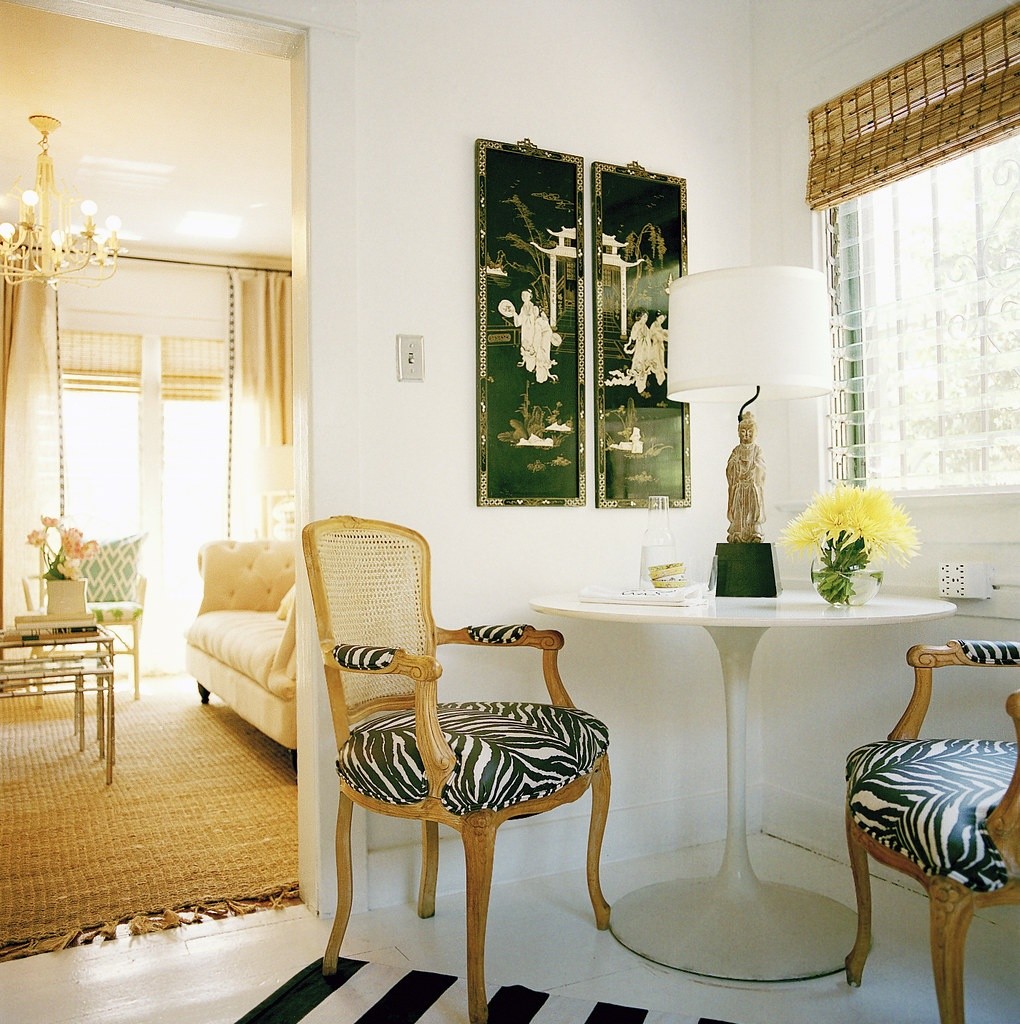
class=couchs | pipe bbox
[184,538,298,776]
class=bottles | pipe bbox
[637,495,679,592]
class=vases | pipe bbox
[810,531,884,607]
[45,579,87,617]
[638,495,675,591]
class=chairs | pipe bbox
[22,531,150,708]
[845,641,1020,1024]
[302,516,614,1023]
[301,515,613,1024]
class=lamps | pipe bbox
[666,264,835,598]
[0,115,128,292]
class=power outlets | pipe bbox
[939,560,992,599]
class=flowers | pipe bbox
[777,485,923,607]
[25,514,100,580]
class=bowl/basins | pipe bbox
[649,566,688,581]
[648,562,685,573]
[651,580,687,591]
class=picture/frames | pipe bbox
[476,137,584,507]
[591,161,693,510]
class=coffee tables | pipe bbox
[0,625,116,784]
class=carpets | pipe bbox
[234,956,737,1024]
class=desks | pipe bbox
[531,588,958,984]
